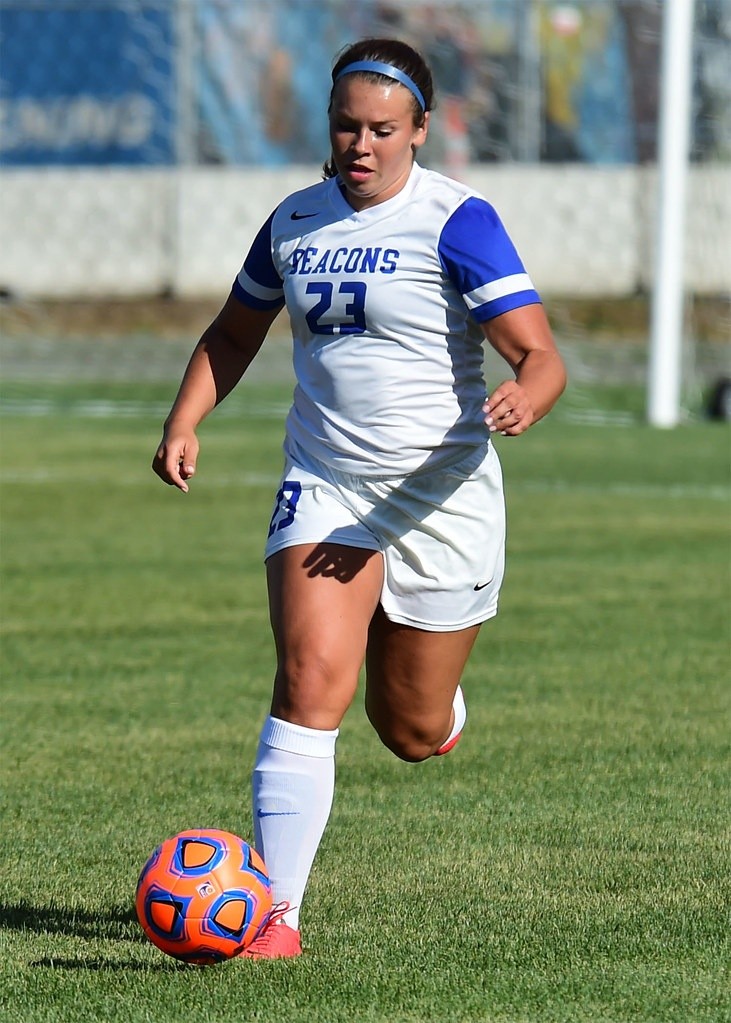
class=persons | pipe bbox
[154,36,567,963]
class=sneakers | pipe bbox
[239,925,304,962]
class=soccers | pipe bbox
[135,830,271,966]
